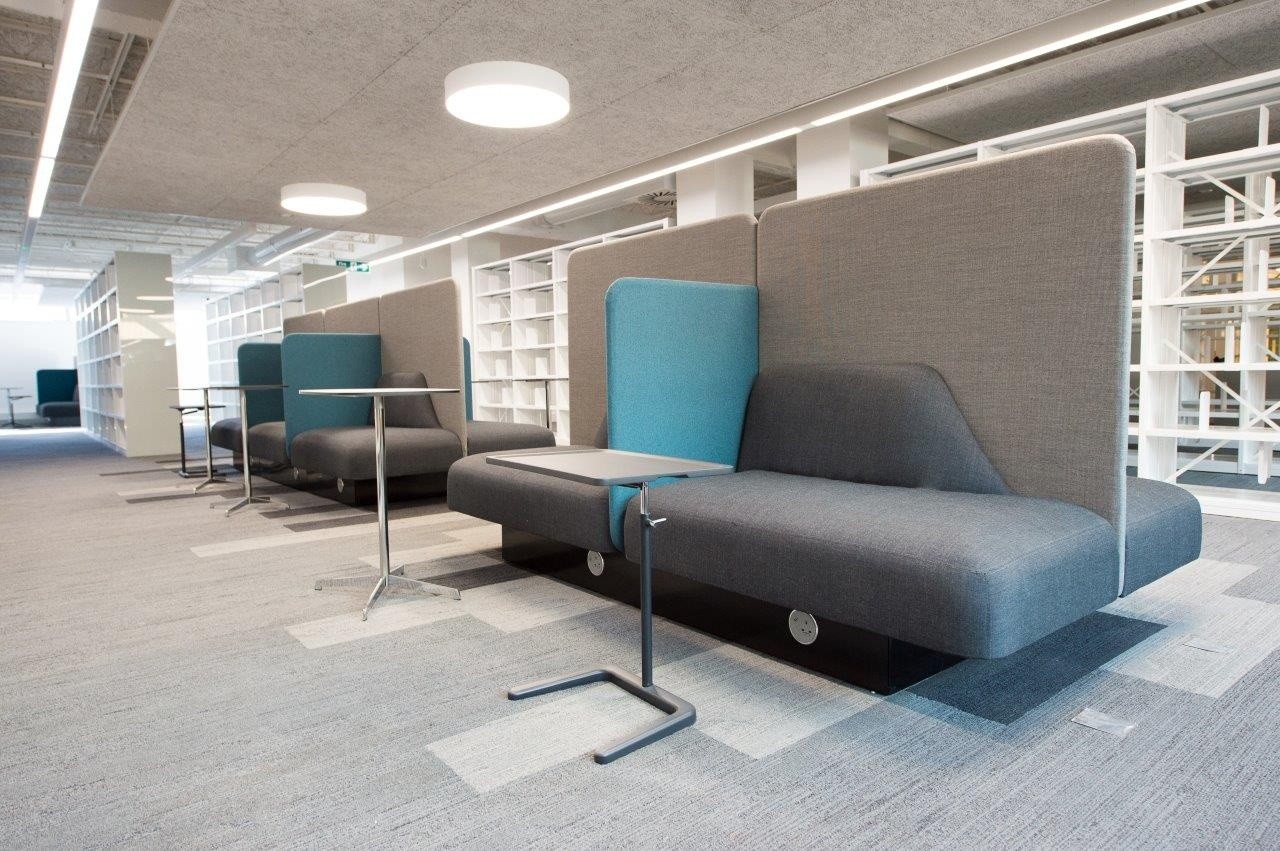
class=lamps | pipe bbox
[281,182,368,217]
[443,60,571,130]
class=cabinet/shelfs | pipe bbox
[469,215,676,445]
[204,262,348,420]
[75,249,183,457]
[857,68,1280,527]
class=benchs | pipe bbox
[466,420,555,456]
[1121,470,1203,600]
[291,371,463,480]
[619,360,1120,697]
[445,444,620,556]
[210,416,244,454]
[245,421,288,465]
[36,368,79,426]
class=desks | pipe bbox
[207,384,292,519]
[167,386,246,494]
[479,447,736,762]
[169,403,230,479]
[296,388,463,620]
[7,393,34,429]
[0,385,25,427]
